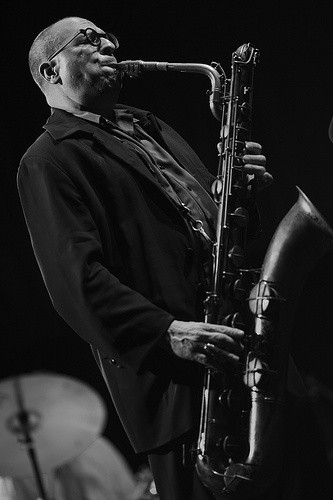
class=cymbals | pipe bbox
[0,373,107,478]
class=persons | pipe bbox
[17,16,333,500]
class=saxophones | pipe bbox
[104,40,333,500]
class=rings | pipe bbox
[203,343,214,354]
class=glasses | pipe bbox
[48,28,119,61]
